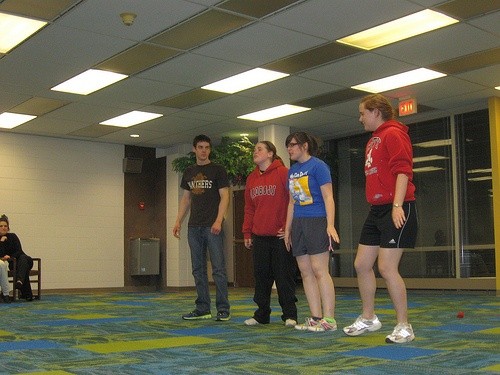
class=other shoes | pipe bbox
[244,317,258,326]
[285,318,296,328]
[15,282,21,289]
[4,295,11,303]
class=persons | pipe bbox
[342,93,415,343]
[241,142,299,327]
[172,135,231,322]
[282,132,341,333]
[0,215,36,303]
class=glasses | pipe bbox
[286,142,304,148]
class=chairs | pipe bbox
[7,258,41,301]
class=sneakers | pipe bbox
[307,318,337,332]
[216,311,232,321]
[343,314,382,336]
[385,323,416,344]
[182,309,212,319]
[295,317,320,331]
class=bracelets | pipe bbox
[392,205,402,207]
